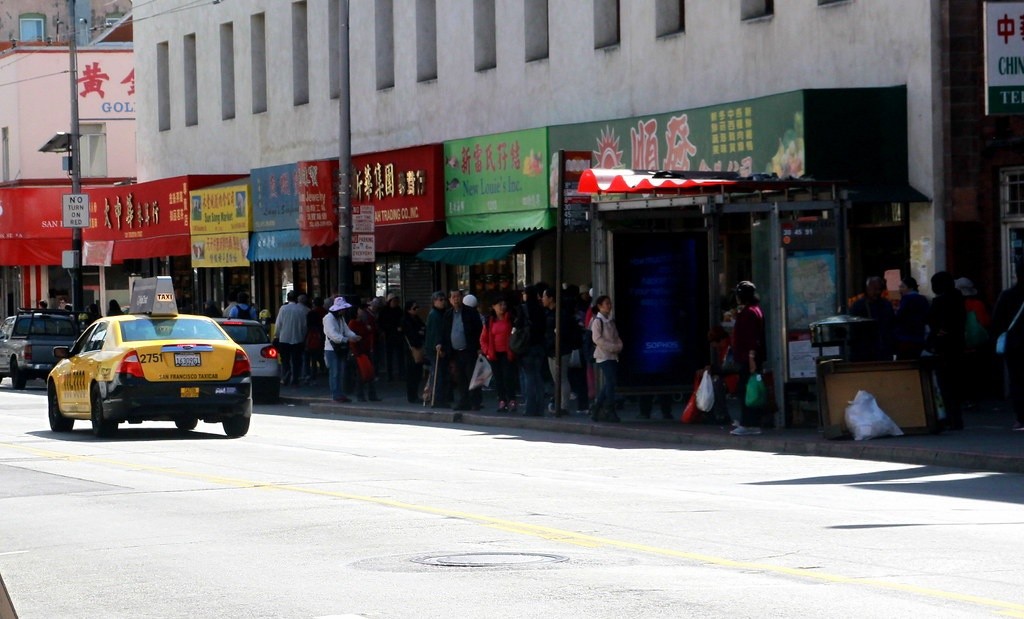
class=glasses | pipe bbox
[59,302,64,304]
[439,298,446,301]
[410,306,419,311]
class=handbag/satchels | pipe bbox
[745,371,766,407]
[680,371,702,423]
[964,311,981,341]
[409,347,423,364]
[509,304,529,355]
[308,331,320,349]
[696,370,714,412]
[581,317,603,362]
[996,331,1007,353]
[468,353,492,390]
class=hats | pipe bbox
[386,293,398,302]
[579,284,590,294]
[204,300,215,306]
[955,278,977,296]
[329,297,352,312]
[731,281,755,293]
[463,295,477,307]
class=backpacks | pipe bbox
[235,305,253,319]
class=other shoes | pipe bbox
[369,396,382,401]
[357,398,367,402]
[496,400,506,412]
[730,425,762,435]
[335,395,352,402]
[411,398,423,403]
[509,400,517,412]
[547,391,674,423]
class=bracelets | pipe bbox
[749,354,755,358]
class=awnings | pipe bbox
[415,227,552,266]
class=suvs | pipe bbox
[210,317,281,400]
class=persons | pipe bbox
[848,275,894,360]
[997,258,1024,430]
[107,299,126,341]
[58,296,70,310]
[38,300,54,334]
[729,281,768,436]
[954,277,989,407]
[927,271,968,425]
[590,295,621,424]
[635,395,675,419]
[88,303,102,321]
[204,280,595,415]
[893,275,928,358]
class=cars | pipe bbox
[48,276,252,440]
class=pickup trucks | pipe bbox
[0,307,81,388]
